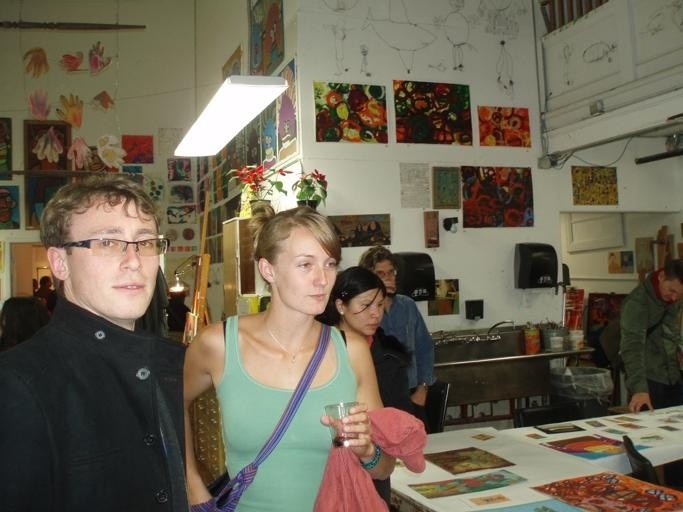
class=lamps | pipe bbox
[165,253,204,346]
[167,0,290,161]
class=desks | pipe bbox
[492,402,682,475]
[381,424,682,511]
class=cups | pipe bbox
[521,327,541,353]
[325,403,360,450]
[549,336,564,352]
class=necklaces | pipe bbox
[263,317,317,366]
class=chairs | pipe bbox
[510,402,583,430]
[620,431,661,485]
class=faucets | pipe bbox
[487,319,514,337]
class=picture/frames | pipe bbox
[21,117,72,233]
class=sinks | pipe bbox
[426,341,596,405]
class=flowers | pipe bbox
[221,157,291,211]
[289,166,331,208]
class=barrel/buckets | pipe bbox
[544,328,566,352]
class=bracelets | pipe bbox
[420,382,428,389]
[361,445,380,471]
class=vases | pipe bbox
[295,197,319,213]
[247,198,271,219]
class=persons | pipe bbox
[32,278,38,293]
[177,198,398,512]
[314,264,433,441]
[0,295,52,354]
[34,275,53,301]
[358,245,437,412]
[613,259,682,415]
[0,171,188,512]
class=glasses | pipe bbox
[59,236,171,257]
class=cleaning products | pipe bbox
[524,321,541,355]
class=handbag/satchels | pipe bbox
[187,464,256,512]
